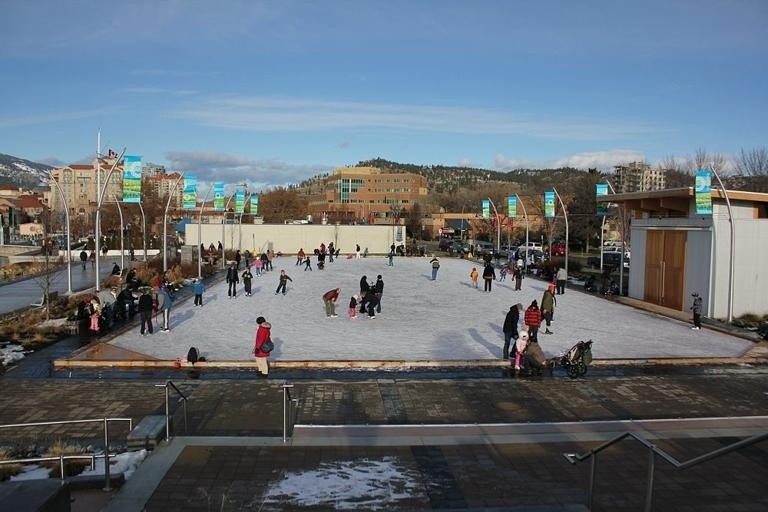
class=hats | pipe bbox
[530,299,539,309]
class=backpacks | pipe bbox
[261,338,275,354]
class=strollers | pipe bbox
[548,339,593,377]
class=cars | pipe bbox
[466,239,570,257]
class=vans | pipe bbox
[604,237,627,247]
[601,247,630,258]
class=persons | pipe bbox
[297,242,339,271]
[253,316,271,376]
[363,248,368,257]
[356,244,361,260]
[691,293,703,330]
[323,288,340,318]
[430,256,440,281]
[388,239,424,265]
[348,275,384,320]
[439,233,567,376]
[44,234,273,337]
[182,347,205,362]
[275,271,292,295]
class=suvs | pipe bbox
[78,234,107,245]
[587,251,630,274]
